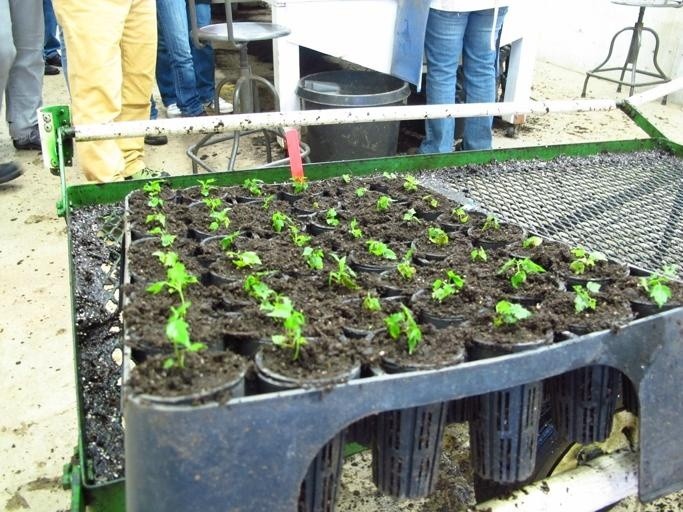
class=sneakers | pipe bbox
[125,166,170,179]
[166,103,181,116]
[208,96,234,115]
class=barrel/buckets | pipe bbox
[294,70,412,163]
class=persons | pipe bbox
[39,1,234,146]
[405,1,508,156]
[0,0,47,192]
[48,0,172,182]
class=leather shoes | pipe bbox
[144,135,167,145]
[0,163,23,184]
[455,141,464,151]
[406,147,420,155]
[13,137,41,150]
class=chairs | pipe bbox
[185,1,313,176]
